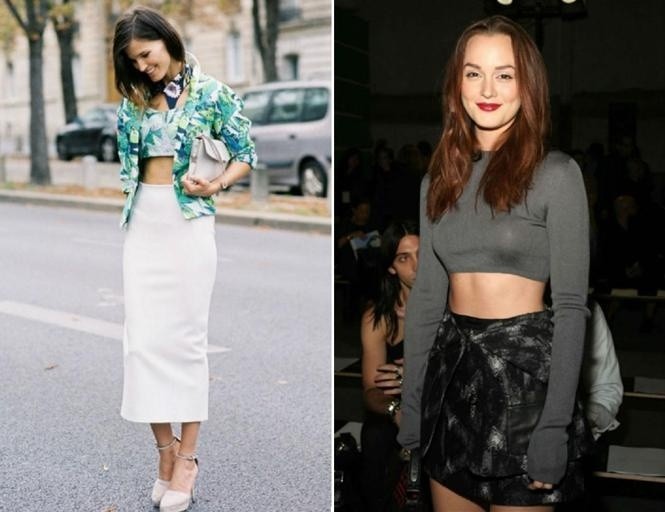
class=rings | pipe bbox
[395,365,401,374]
[397,375,405,385]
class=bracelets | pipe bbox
[217,174,232,191]
[383,397,402,417]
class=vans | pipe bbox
[220,80,330,196]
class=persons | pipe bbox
[393,9,599,510]
[551,280,628,510]
[360,222,420,510]
[334,134,665,335]
[112,6,259,512]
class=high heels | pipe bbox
[151,436,198,512]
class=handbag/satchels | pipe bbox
[186,134,230,186]
[391,450,423,511]
[334,432,365,511]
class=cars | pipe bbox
[57,107,119,162]
